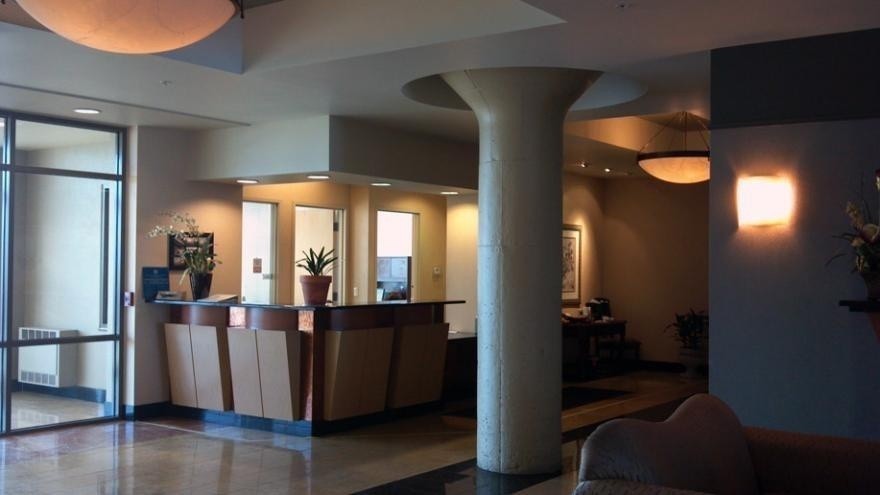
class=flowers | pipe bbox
[820,169,880,309]
[140,204,232,283]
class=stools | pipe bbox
[600,340,642,363]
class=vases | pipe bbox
[190,271,210,301]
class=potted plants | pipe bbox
[663,310,705,366]
[295,245,339,306]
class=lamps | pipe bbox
[632,109,714,185]
[18,0,241,57]
[731,157,802,243]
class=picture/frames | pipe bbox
[558,223,583,307]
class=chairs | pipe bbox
[573,389,880,495]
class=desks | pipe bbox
[561,319,629,374]
